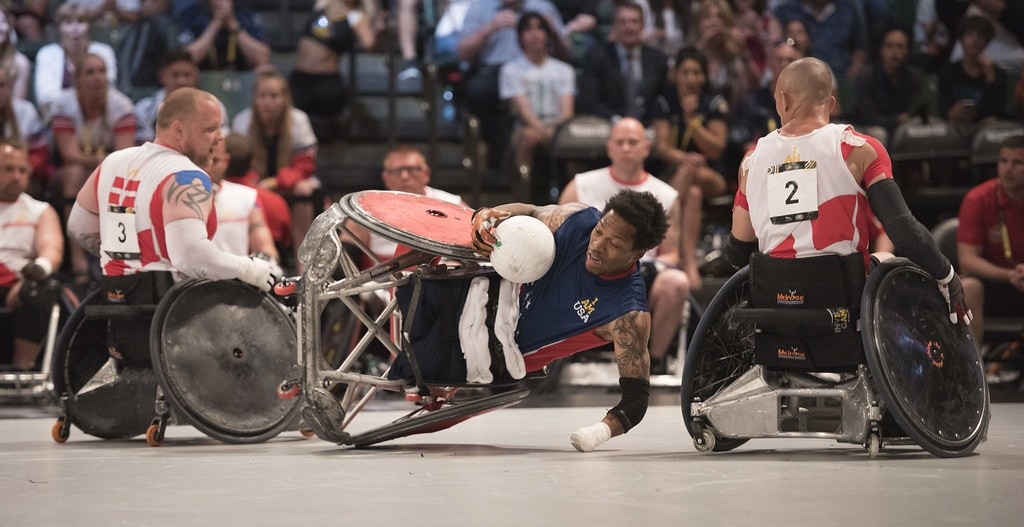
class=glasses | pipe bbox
[383,164,426,179]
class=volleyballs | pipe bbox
[487,214,555,284]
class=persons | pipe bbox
[386,189,671,452]
[430,0,1024,376]
[726,57,973,324]
[0,0,469,390]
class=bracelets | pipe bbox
[229,25,242,33]
[935,264,954,284]
[34,257,53,275]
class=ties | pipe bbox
[625,52,641,122]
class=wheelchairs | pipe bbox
[269,190,549,450]
[1,287,81,404]
[47,264,316,448]
[680,254,992,459]
[534,281,703,395]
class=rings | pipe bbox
[478,223,485,234]
[473,239,478,246]
